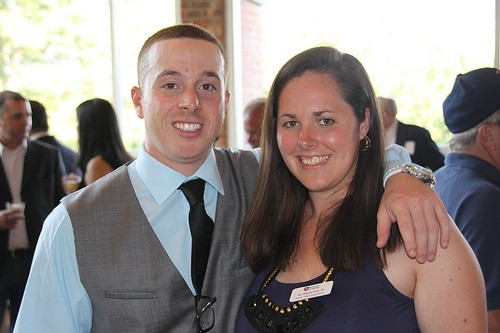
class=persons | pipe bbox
[12,24,453,333]
[200,46,488,333]
[0,68,500,333]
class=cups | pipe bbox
[6,204,25,224]
[63,175,82,195]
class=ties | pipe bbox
[177,178,215,295]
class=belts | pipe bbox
[7,249,34,257]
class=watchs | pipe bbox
[382,164,436,187]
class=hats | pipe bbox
[443,68,500,133]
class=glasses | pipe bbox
[193,295,216,333]
